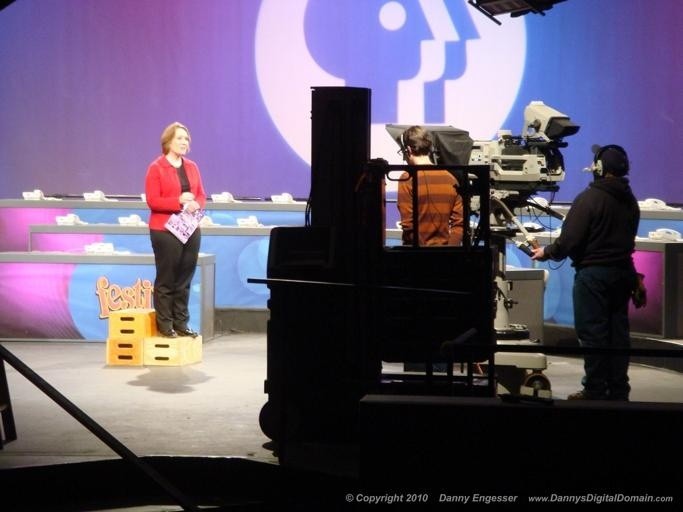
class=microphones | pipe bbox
[583,166,599,172]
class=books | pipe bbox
[164,204,204,244]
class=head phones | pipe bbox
[591,148,605,177]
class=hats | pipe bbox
[591,144,630,178]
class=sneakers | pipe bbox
[567,388,630,402]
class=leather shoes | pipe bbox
[159,327,197,338]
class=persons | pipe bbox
[397,125,464,246]
[145,122,207,337]
[531,143,640,402]
